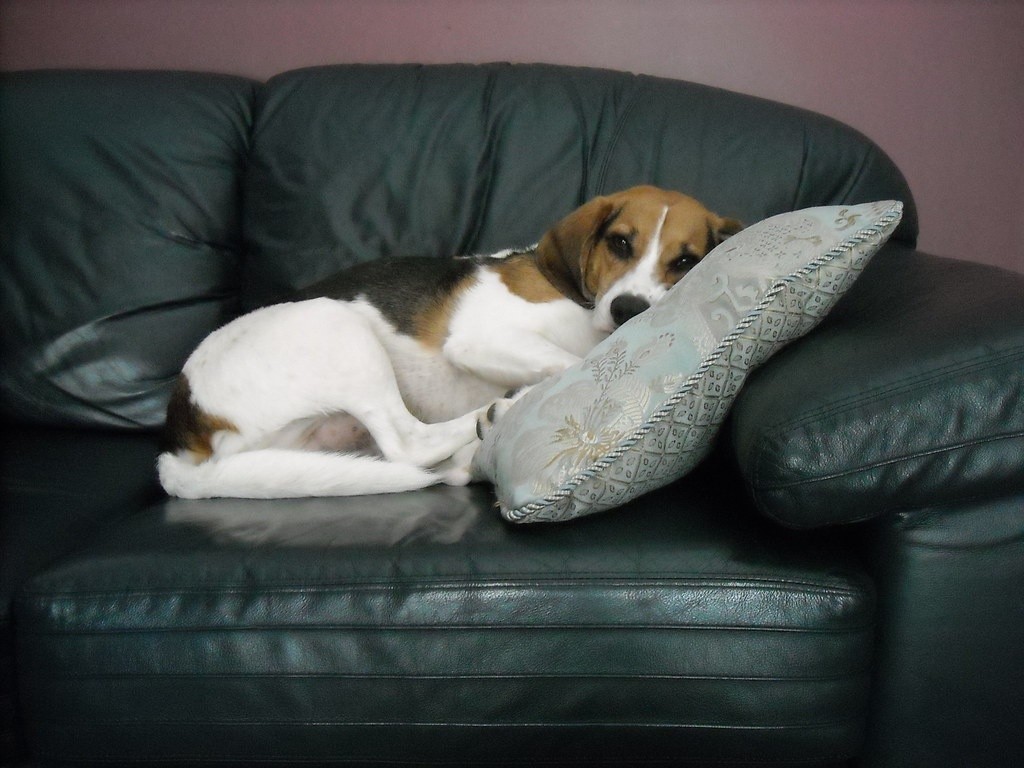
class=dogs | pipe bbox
[154,184,744,501]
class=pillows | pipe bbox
[471,201,905,524]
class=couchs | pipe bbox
[0,59,1024,768]
